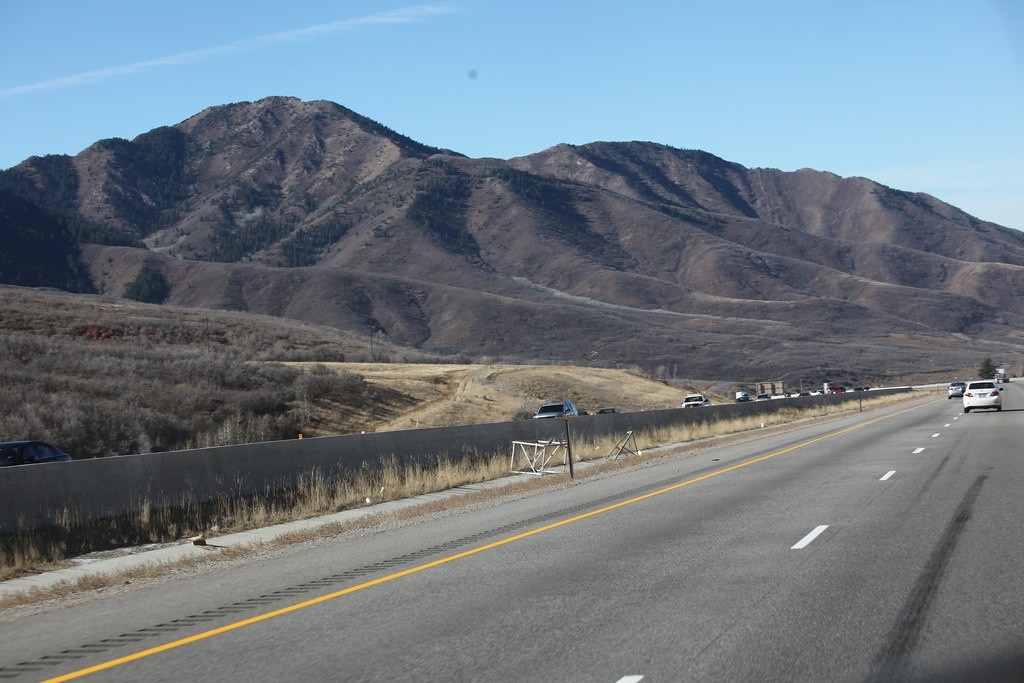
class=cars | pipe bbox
[736,396,754,403]
[755,393,770,402]
[832,386,846,394]
[997,374,1009,383]
[962,380,1004,413]
[0,441,72,466]
[947,382,968,399]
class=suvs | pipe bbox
[532,401,578,418]
[680,394,708,408]
